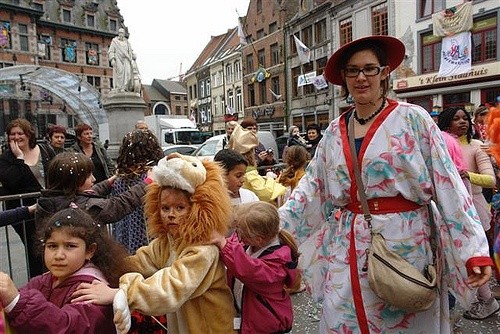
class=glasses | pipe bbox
[343,67,385,78]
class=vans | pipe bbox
[190,130,281,177]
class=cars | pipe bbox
[36,130,76,148]
[162,145,212,158]
[274,135,289,158]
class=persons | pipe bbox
[71,154,235,334]
[426,103,500,321]
[217,119,323,209]
[135,121,148,130]
[0,207,131,334]
[28,153,154,280]
[275,35,493,334]
[48,124,66,156]
[108,28,136,93]
[66,124,114,185]
[0,120,55,282]
[200,201,298,334]
[0,203,38,228]
[111,129,165,256]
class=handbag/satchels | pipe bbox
[363,233,441,315]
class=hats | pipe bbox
[324,35,405,84]
[228,124,260,154]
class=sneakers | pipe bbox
[463,294,500,320]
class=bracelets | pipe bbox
[145,177,154,184]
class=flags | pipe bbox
[431,1,473,35]
[238,16,247,44]
[293,36,310,64]
[437,32,472,77]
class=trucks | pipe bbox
[144,114,205,147]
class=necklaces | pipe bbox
[353,96,386,124]
